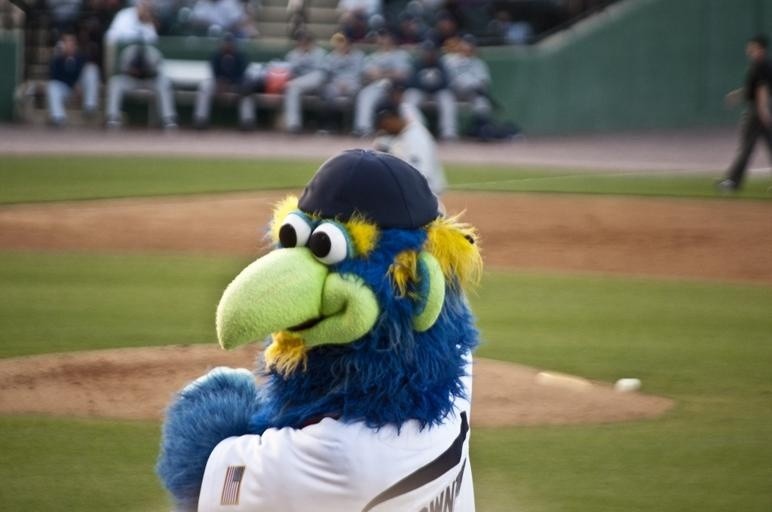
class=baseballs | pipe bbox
[614,377,641,394]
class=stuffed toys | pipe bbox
[156,148,483,512]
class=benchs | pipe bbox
[0,0,475,137]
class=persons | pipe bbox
[713,37,771,190]
[373,97,448,196]
[1,0,493,138]
